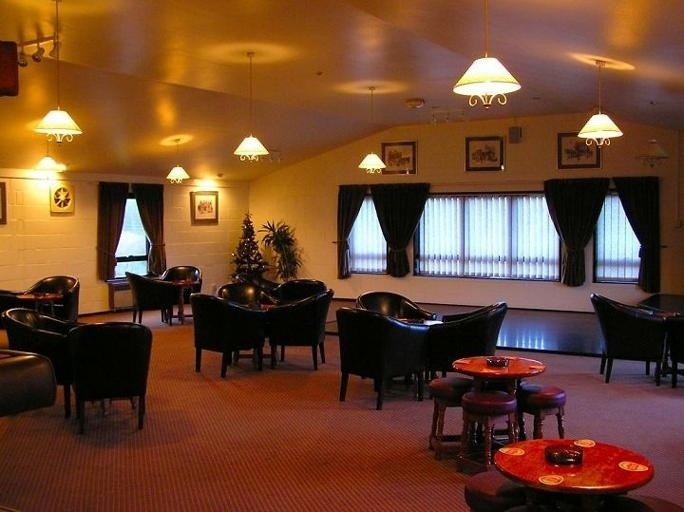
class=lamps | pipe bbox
[18,42,30,68]
[452,1,522,110]
[635,99,669,167]
[165,139,190,186]
[31,40,45,62]
[576,60,624,151]
[35,0,83,144]
[358,87,387,176]
[234,52,269,167]
[50,37,62,61]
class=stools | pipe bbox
[591,493,684,512]
[464,470,526,512]
[456,390,518,472]
[516,384,566,439]
[428,377,472,460]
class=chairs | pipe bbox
[271,279,328,301]
[190,292,266,377]
[641,295,683,316]
[126,272,177,326]
[592,293,665,384]
[266,288,334,370]
[24,275,80,322]
[66,322,153,429]
[158,266,202,318]
[0,290,21,329]
[336,306,429,412]
[216,284,274,300]
[666,309,684,384]
[6,307,72,417]
[430,303,506,388]
[356,291,431,325]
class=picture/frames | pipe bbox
[557,133,601,169]
[464,135,504,171]
[190,191,218,223]
[381,142,416,175]
[1,181,7,226]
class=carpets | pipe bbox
[1,301,683,512]
